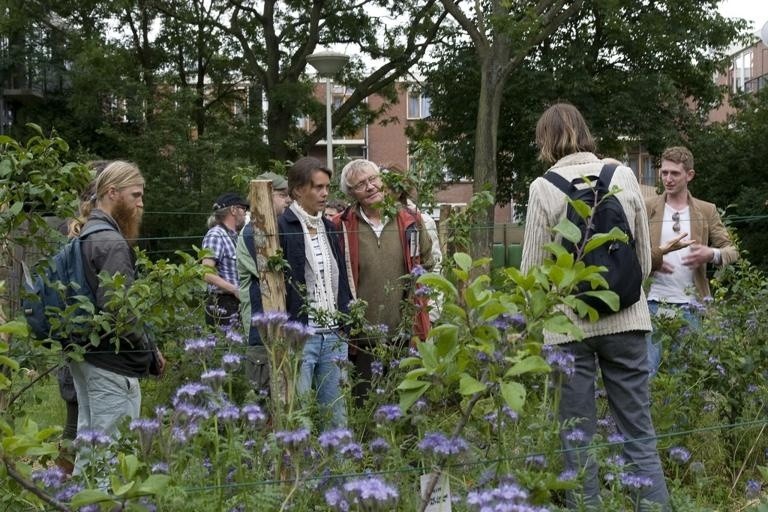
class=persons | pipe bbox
[323,199,349,222]
[201,192,250,373]
[57,180,100,439]
[519,102,674,512]
[331,158,437,408]
[235,171,293,393]
[64,158,168,478]
[378,160,445,322]
[276,155,355,429]
[643,145,739,401]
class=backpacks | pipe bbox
[540,165,642,321]
[22,236,97,349]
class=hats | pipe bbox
[212,193,251,212]
[250,172,289,190]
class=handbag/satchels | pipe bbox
[203,292,241,333]
